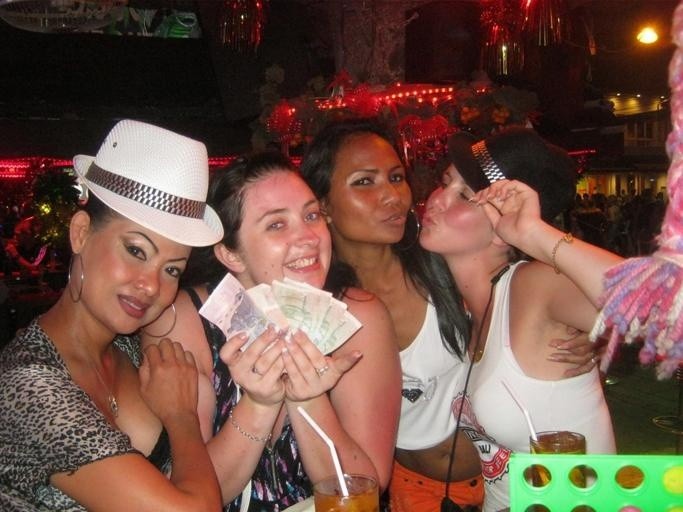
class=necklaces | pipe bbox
[470,343,487,362]
[92,350,120,417]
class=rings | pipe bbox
[588,352,595,359]
[592,355,600,364]
[317,364,330,376]
[250,367,264,376]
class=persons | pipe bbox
[562,185,666,257]
[0,119,225,512]
[300,114,608,512]
[1,179,65,297]
[137,151,403,512]
[419,125,630,511]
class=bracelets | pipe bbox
[551,231,573,275]
[229,406,273,441]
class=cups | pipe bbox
[531,431,585,512]
[313,472,379,512]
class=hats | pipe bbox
[71,118,226,248]
[449,123,575,226]
